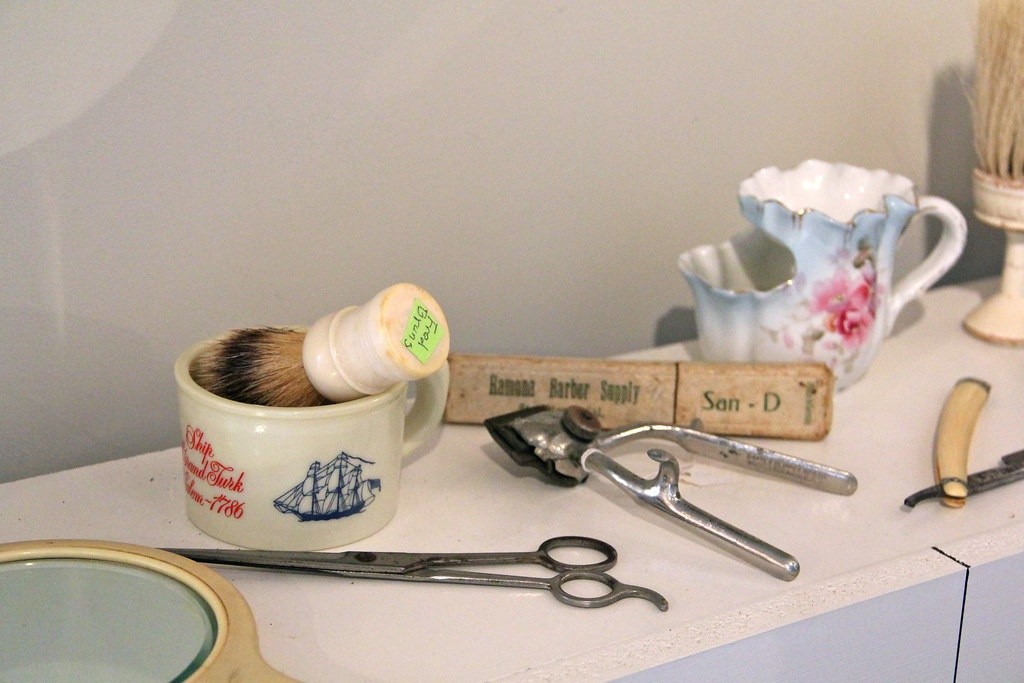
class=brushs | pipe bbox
[189,278,452,408]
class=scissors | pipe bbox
[155,536,672,614]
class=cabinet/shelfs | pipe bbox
[0,274,1024,683]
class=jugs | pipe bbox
[737,156,972,393]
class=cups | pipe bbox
[174,329,456,551]
[678,229,794,362]
